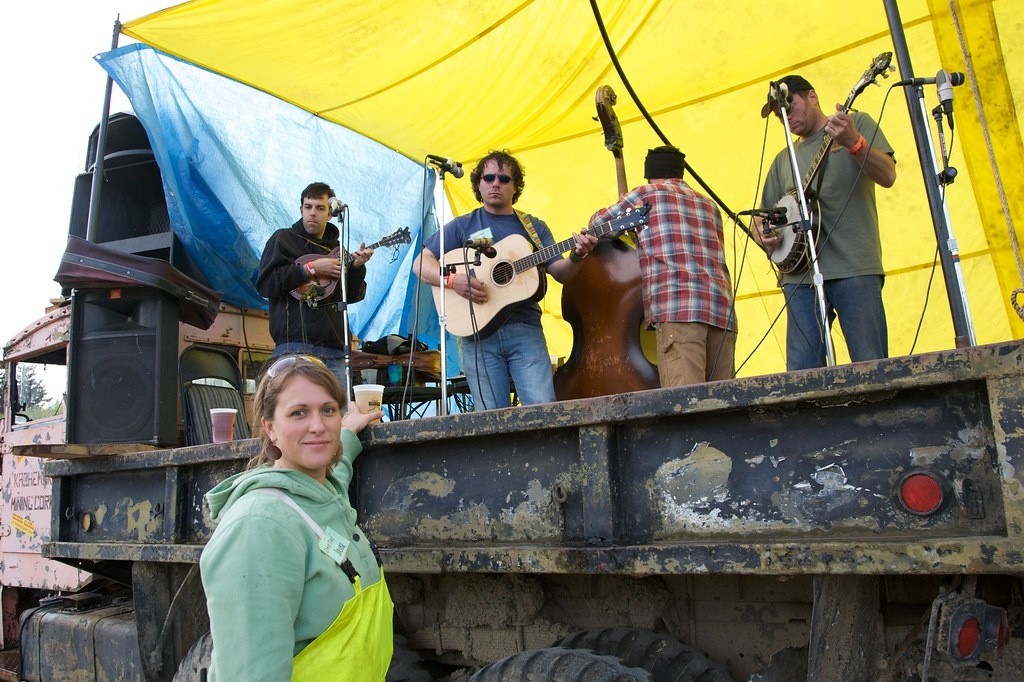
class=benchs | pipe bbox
[382,386,452,423]
[448,376,519,413]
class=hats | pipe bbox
[761,75,814,119]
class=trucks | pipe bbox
[0,110,1022,682]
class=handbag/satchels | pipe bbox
[363,333,428,355]
[56,235,223,331]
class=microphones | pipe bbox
[429,159,464,178]
[300,282,317,300]
[771,82,794,103]
[776,84,792,115]
[330,205,345,217]
[464,238,493,245]
[892,72,965,87]
[470,244,497,258]
[739,207,787,215]
[754,211,788,224]
[935,69,954,130]
[327,197,348,208]
[428,154,462,167]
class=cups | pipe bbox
[209,408,238,443]
[355,384,385,423]
[361,370,378,384]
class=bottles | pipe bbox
[387,360,403,386]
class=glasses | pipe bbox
[480,174,514,183]
[266,353,327,378]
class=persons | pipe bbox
[412,150,598,412]
[752,75,897,372]
[199,353,395,682]
[589,145,738,389]
[255,182,373,417]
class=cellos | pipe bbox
[551,85,661,402]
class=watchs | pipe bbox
[569,250,588,264]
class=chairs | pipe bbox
[178,344,252,447]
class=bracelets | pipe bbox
[307,262,316,275]
[444,273,456,289]
[847,134,866,155]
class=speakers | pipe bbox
[64,287,179,444]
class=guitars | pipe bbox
[430,207,650,342]
[769,50,896,275]
[285,227,412,304]
